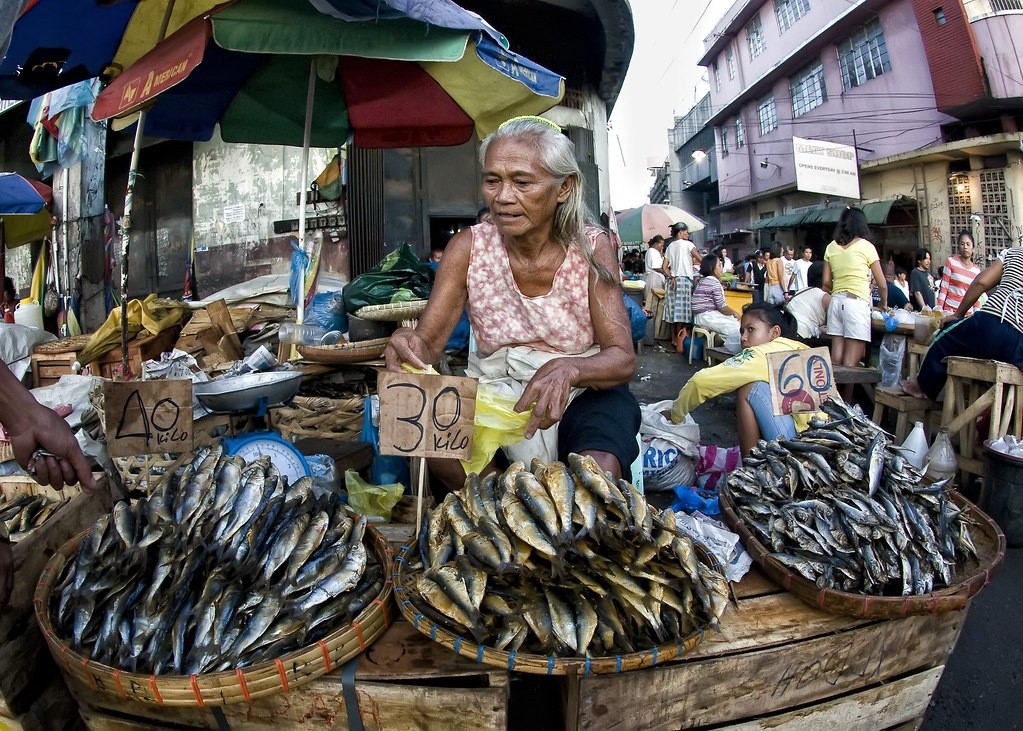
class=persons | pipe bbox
[384,122,642,501]
[692,253,741,346]
[0,289,17,324]
[822,207,887,366]
[978,290,989,308]
[784,262,833,355]
[900,245,1023,400]
[911,249,935,311]
[938,232,980,317]
[0,358,97,494]
[662,222,703,345]
[712,240,812,304]
[873,262,944,312]
[658,302,845,467]
[643,234,665,339]
[622,249,647,274]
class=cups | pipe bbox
[61,324,67,336]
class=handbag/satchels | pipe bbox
[696,443,742,490]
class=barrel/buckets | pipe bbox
[980,439,1023,548]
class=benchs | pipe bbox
[702,345,968,450]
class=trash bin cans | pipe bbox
[980,439,1023,548]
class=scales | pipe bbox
[194,370,311,485]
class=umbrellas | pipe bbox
[615,203,708,244]
[0,0,565,375]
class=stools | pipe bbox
[688,325,717,367]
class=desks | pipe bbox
[870,312,941,381]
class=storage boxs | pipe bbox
[61,540,973,731]
[723,292,753,316]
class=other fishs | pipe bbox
[0,491,71,543]
[417,453,732,656]
[51,442,367,676]
[727,395,979,596]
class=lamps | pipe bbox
[760,157,779,169]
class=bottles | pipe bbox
[676,328,703,361]
[923,426,957,489]
[4,309,15,324]
[278,323,326,346]
[14,298,43,331]
[899,422,929,470]
